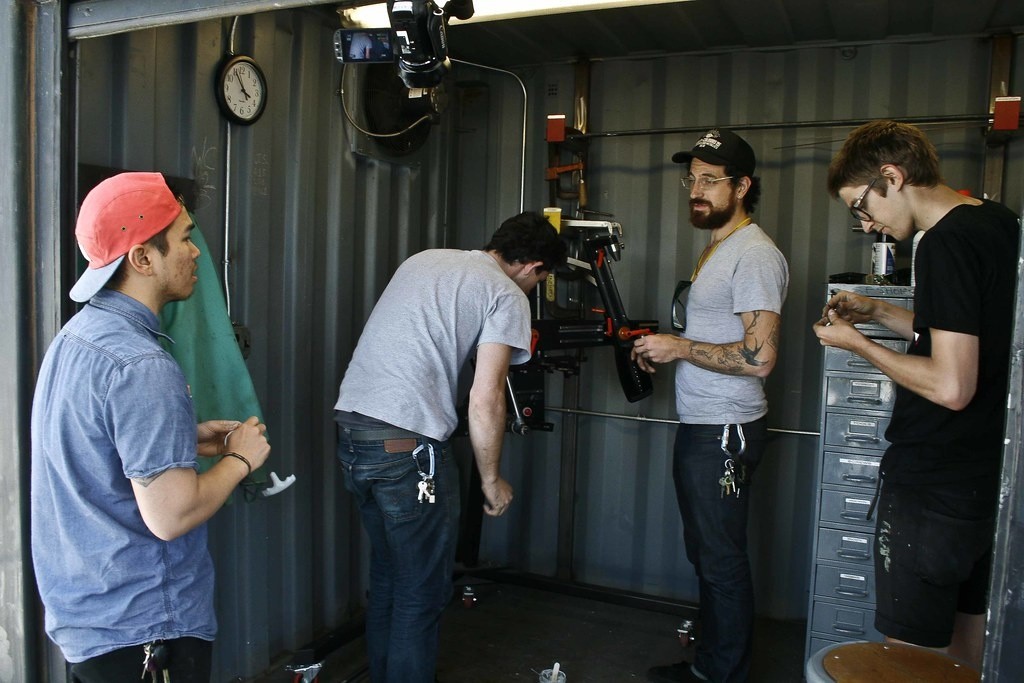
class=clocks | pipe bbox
[215,54,268,125]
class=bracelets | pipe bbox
[222,451,252,478]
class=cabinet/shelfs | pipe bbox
[801,283,914,668]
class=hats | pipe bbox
[671,127,756,177]
[68,171,184,304]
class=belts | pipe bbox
[334,426,425,440]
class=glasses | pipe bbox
[851,178,877,222]
[671,281,691,332]
[681,177,735,188]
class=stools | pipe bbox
[806,639,981,683]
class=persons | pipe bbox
[811,120,1022,657]
[32,171,271,683]
[349,31,389,61]
[630,131,789,682]
[334,211,560,682]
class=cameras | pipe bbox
[331,0,474,88]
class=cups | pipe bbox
[538,668,567,683]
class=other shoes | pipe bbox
[648,662,709,683]
